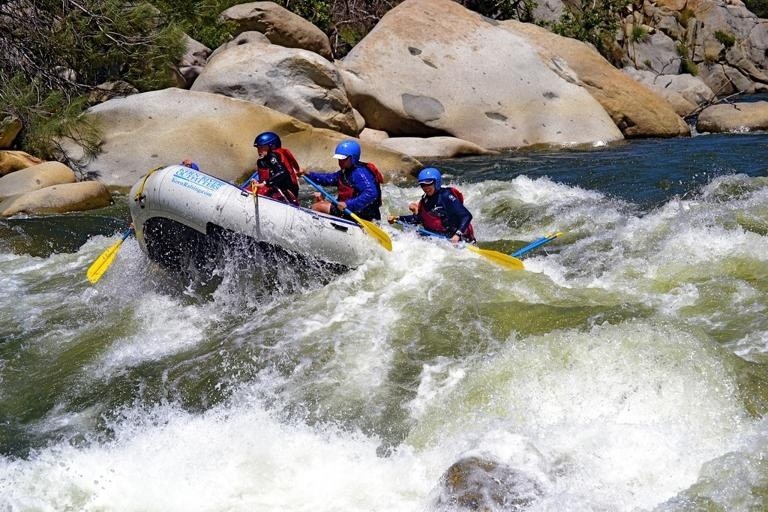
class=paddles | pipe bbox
[86,229,131,284]
[300,172,391,252]
[252,182,257,197]
[393,220,524,272]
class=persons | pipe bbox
[387,168,477,246]
[245,132,300,207]
[129,160,199,231]
[409,202,419,215]
[301,140,384,224]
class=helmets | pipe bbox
[417,167,441,192]
[332,140,361,165]
[253,131,282,150]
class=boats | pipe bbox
[128,163,399,288]
[721,131,755,136]
